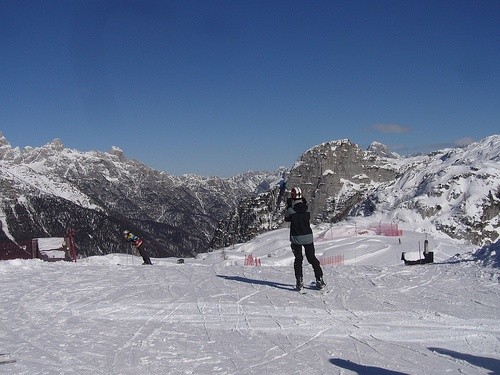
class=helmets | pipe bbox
[291,188,302,200]
[123,230,129,234]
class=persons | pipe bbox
[284,198,325,289]
[124,231,151,265]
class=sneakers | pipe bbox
[295,277,304,294]
[316,277,326,291]
[142,262,153,265]
